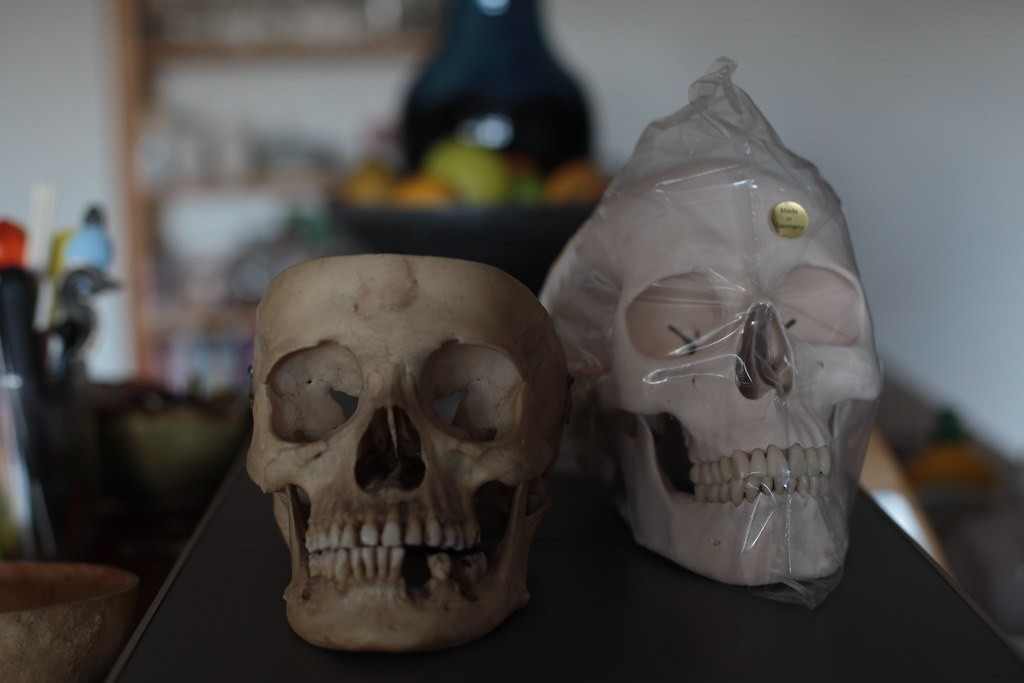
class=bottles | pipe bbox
[64,207,111,273]
[400,0,593,182]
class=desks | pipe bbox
[105,406,1024,683]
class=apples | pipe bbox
[424,142,550,203]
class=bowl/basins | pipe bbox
[330,196,597,287]
[1,562,142,683]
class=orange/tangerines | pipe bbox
[341,162,604,206]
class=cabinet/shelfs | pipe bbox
[113,0,441,381]
